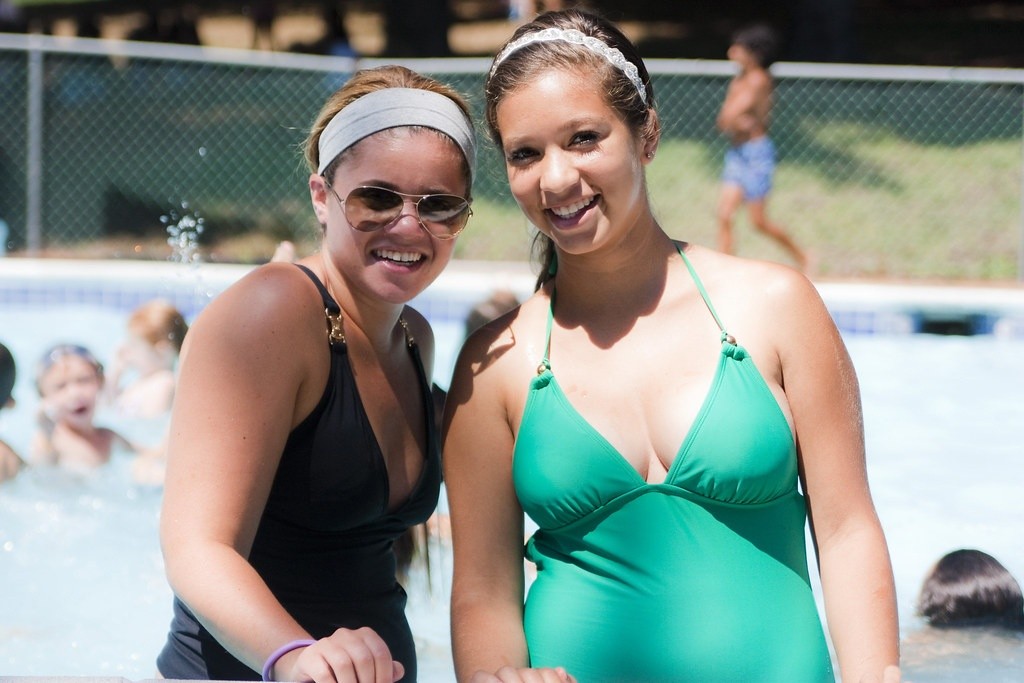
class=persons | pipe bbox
[715,26,811,274]
[444,10,900,682]
[151,63,478,683]
[916,549,1024,641]
[0,300,522,561]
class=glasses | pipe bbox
[325,180,473,241]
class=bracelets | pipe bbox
[263,639,320,681]
